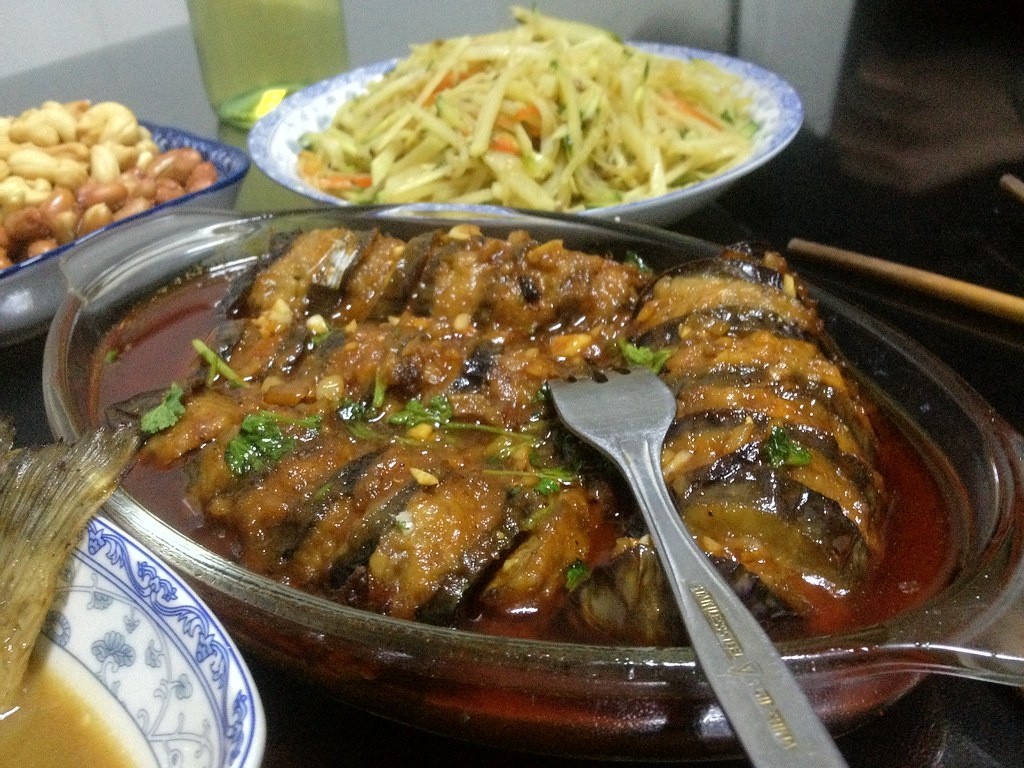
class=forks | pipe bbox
[545,361,853,767]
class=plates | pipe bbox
[249,39,806,233]
[0,118,254,352]
[1,510,268,768]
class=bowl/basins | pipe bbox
[43,198,1023,754]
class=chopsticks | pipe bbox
[784,237,1023,324]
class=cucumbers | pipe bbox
[314,7,747,211]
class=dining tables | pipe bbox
[4,0,1024,768]
